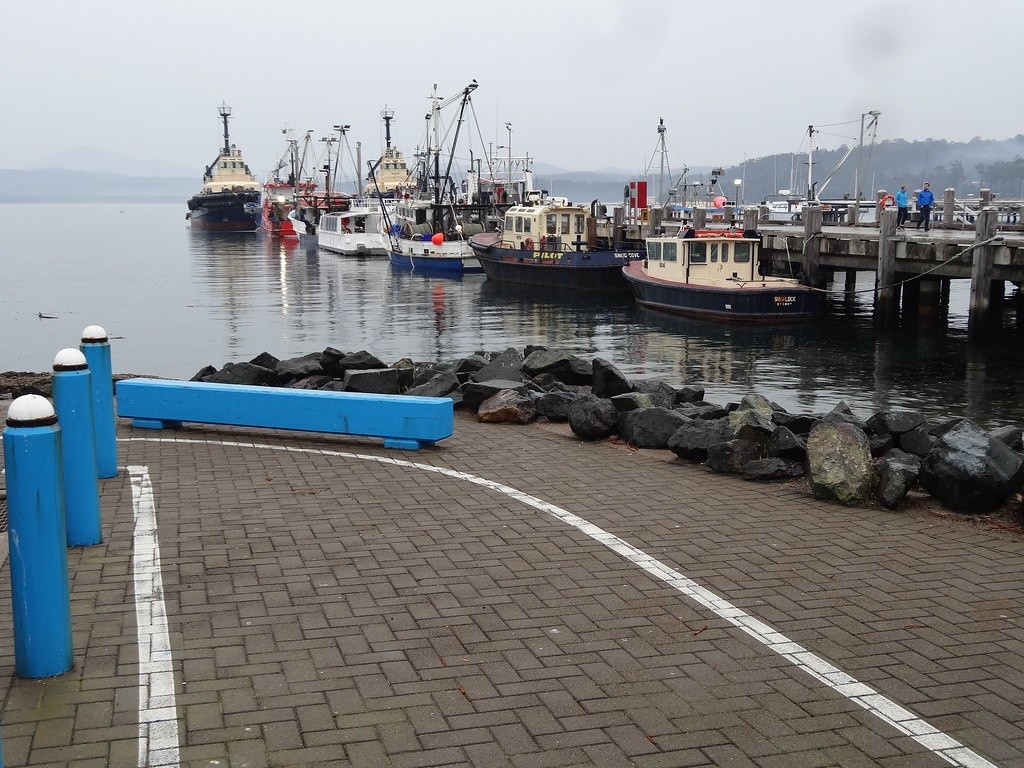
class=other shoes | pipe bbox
[923,228,930,232]
[916,224,920,229]
[897,223,905,232]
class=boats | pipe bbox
[622,214,838,322]
[185,100,263,232]
[469,195,647,287]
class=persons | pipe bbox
[917,182,934,230]
[894,185,908,231]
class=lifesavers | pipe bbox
[880,194,896,209]
[185,212,190,219]
[523,237,534,251]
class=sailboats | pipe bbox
[258,79,549,267]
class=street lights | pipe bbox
[734,179,742,206]
